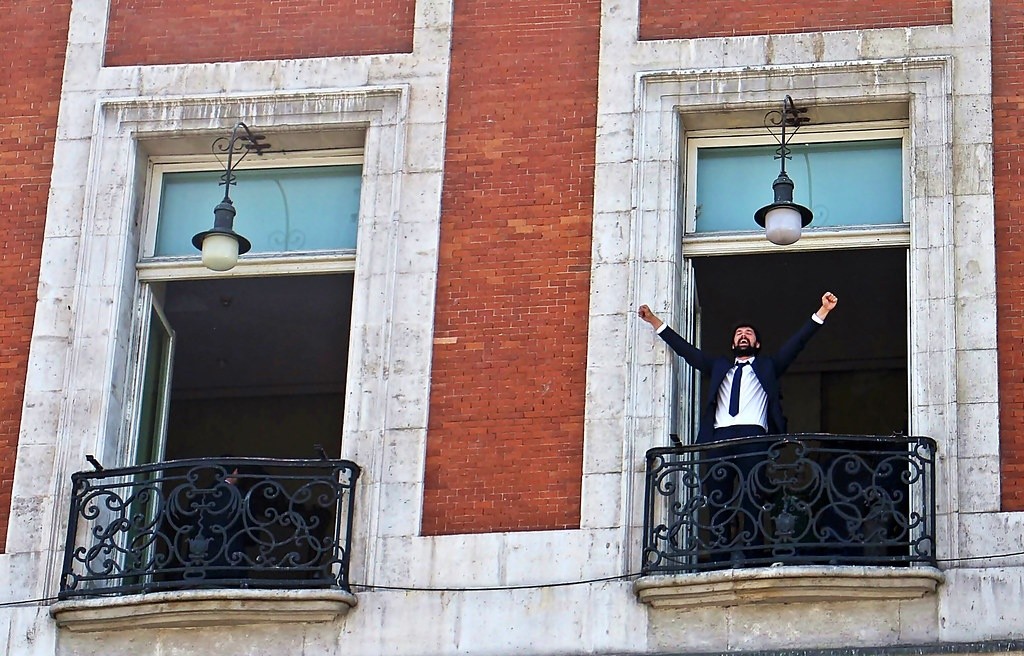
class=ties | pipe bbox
[729,362,750,417]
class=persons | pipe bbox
[185,453,287,589]
[638,292,838,571]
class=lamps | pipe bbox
[752,94,813,246]
[190,121,272,273]
